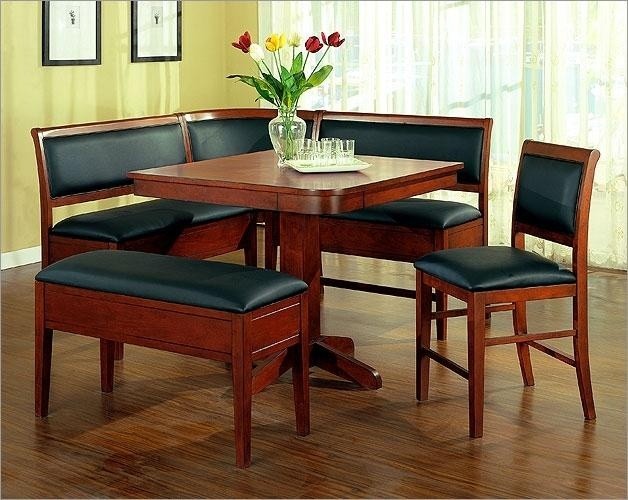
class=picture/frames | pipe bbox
[130,1,183,63]
[38,0,103,66]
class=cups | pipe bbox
[292,137,355,165]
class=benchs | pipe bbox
[293,106,503,348]
[24,102,261,369]
[25,248,315,470]
[168,100,330,310]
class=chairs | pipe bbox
[409,134,602,442]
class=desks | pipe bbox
[115,139,475,405]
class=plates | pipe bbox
[283,156,372,175]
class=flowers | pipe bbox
[220,25,350,167]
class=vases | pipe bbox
[266,110,310,171]
